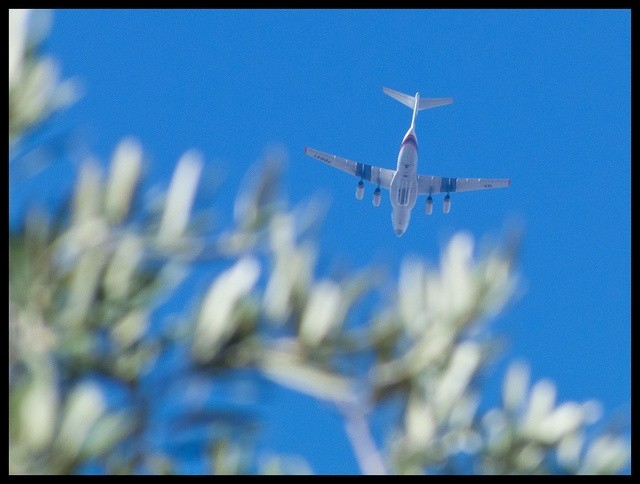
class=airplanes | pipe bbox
[300,86,513,239]
